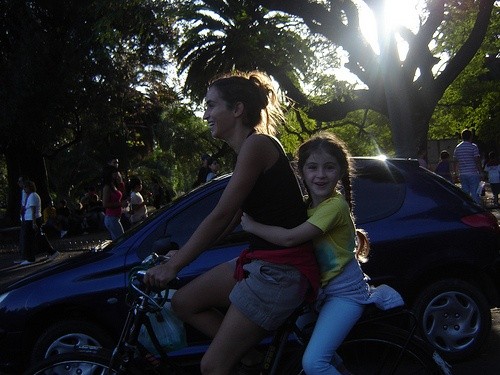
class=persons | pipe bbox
[436,150,454,185]
[241,134,405,375]
[142,72,316,375]
[484,151,500,205]
[206,157,222,182]
[12,157,149,265]
[453,129,483,205]
[198,153,210,186]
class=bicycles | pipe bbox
[22,253,458,375]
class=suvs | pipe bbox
[0,156,500,375]
